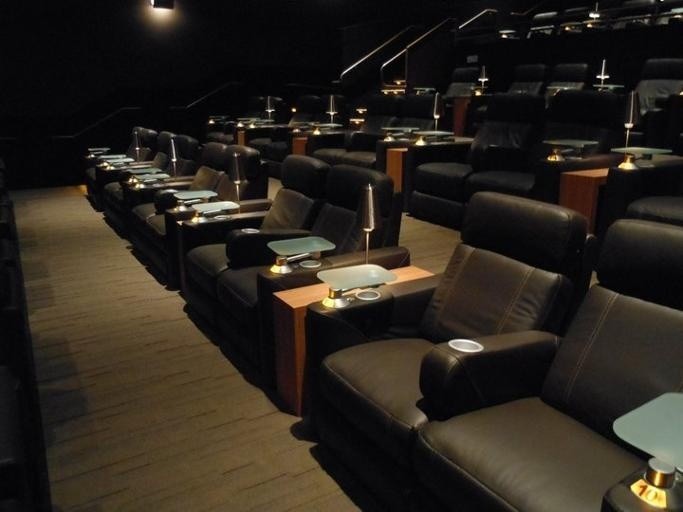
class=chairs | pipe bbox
[308,187,681,506]
[189,58,682,234]
[83,124,407,419]
